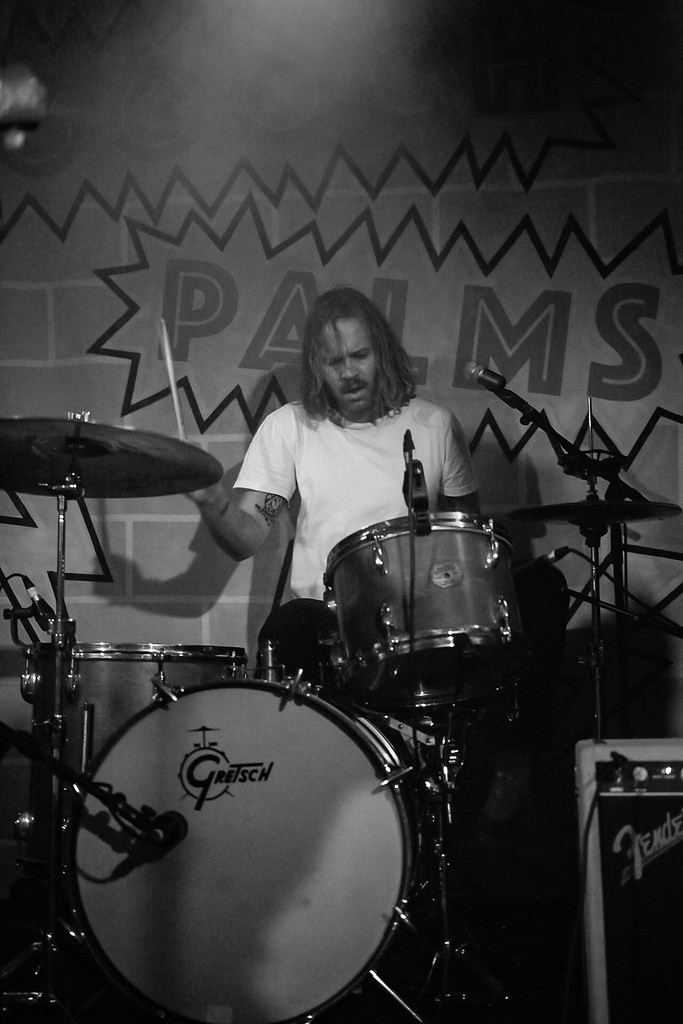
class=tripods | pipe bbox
[0,476,91,1024]
[410,711,527,1024]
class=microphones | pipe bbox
[111,810,189,880]
[462,363,528,409]
[510,546,571,581]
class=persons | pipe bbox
[181,286,481,611]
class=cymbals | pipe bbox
[0,417,224,499]
[505,496,683,528]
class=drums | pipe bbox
[322,512,519,708]
[33,640,249,804]
[63,677,434,1024]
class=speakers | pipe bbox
[574,737,683,1024]
